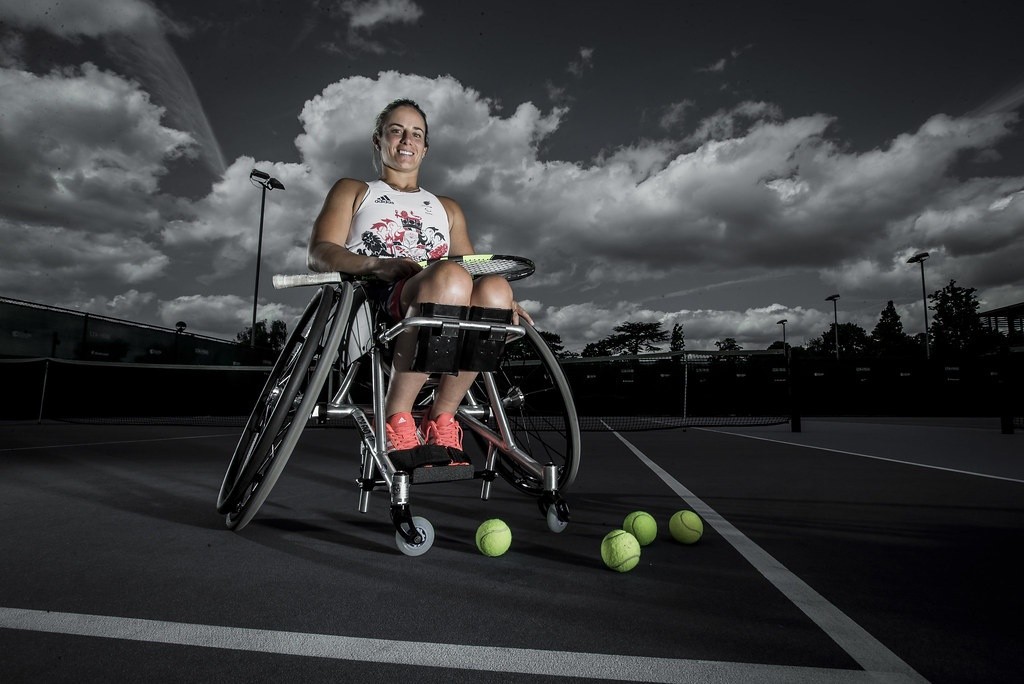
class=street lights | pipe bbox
[907,251,932,363]
[247,167,285,349]
[775,319,787,374]
[825,294,842,360]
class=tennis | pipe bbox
[621,510,661,548]
[600,529,643,573]
[670,510,705,544]
[475,518,512,558]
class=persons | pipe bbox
[306,99,535,469]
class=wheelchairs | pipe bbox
[215,273,581,557]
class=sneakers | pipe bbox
[416,408,470,465]
[372,413,434,468]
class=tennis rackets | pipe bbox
[272,254,538,290]
